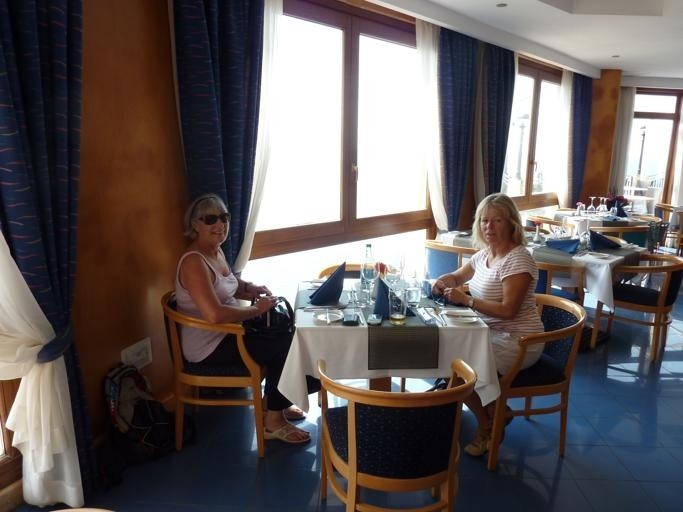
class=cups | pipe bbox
[404,288,421,309]
[385,261,401,283]
[388,289,406,326]
[353,282,368,308]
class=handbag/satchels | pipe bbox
[243,297,293,333]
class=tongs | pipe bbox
[423,306,448,328]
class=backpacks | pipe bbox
[102,362,173,457]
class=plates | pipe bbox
[446,309,478,324]
[313,312,342,322]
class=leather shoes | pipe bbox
[487,406,513,451]
[464,441,488,456]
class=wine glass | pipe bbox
[550,216,591,243]
[575,196,618,218]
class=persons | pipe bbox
[430,191,548,463]
[176,193,310,446]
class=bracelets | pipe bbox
[465,295,477,309]
[242,282,251,293]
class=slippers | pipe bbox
[263,405,305,420]
[264,423,310,443]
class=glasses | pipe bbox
[199,213,232,225]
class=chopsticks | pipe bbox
[325,308,329,325]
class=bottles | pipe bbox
[361,244,376,283]
